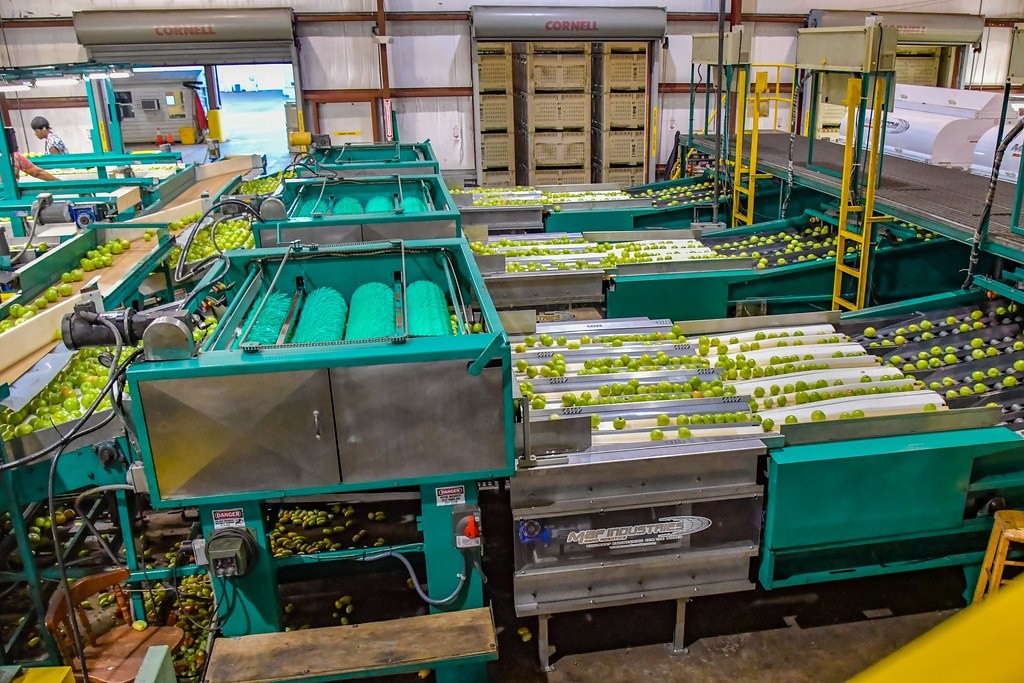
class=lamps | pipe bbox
[0,74,83,92]
[89,69,135,79]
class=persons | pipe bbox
[31,116,68,153]
[14,153,58,181]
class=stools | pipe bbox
[971,510,1024,604]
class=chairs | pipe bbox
[45,569,185,683]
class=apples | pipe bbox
[0,151,1024,683]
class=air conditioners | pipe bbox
[141,100,158,110]
